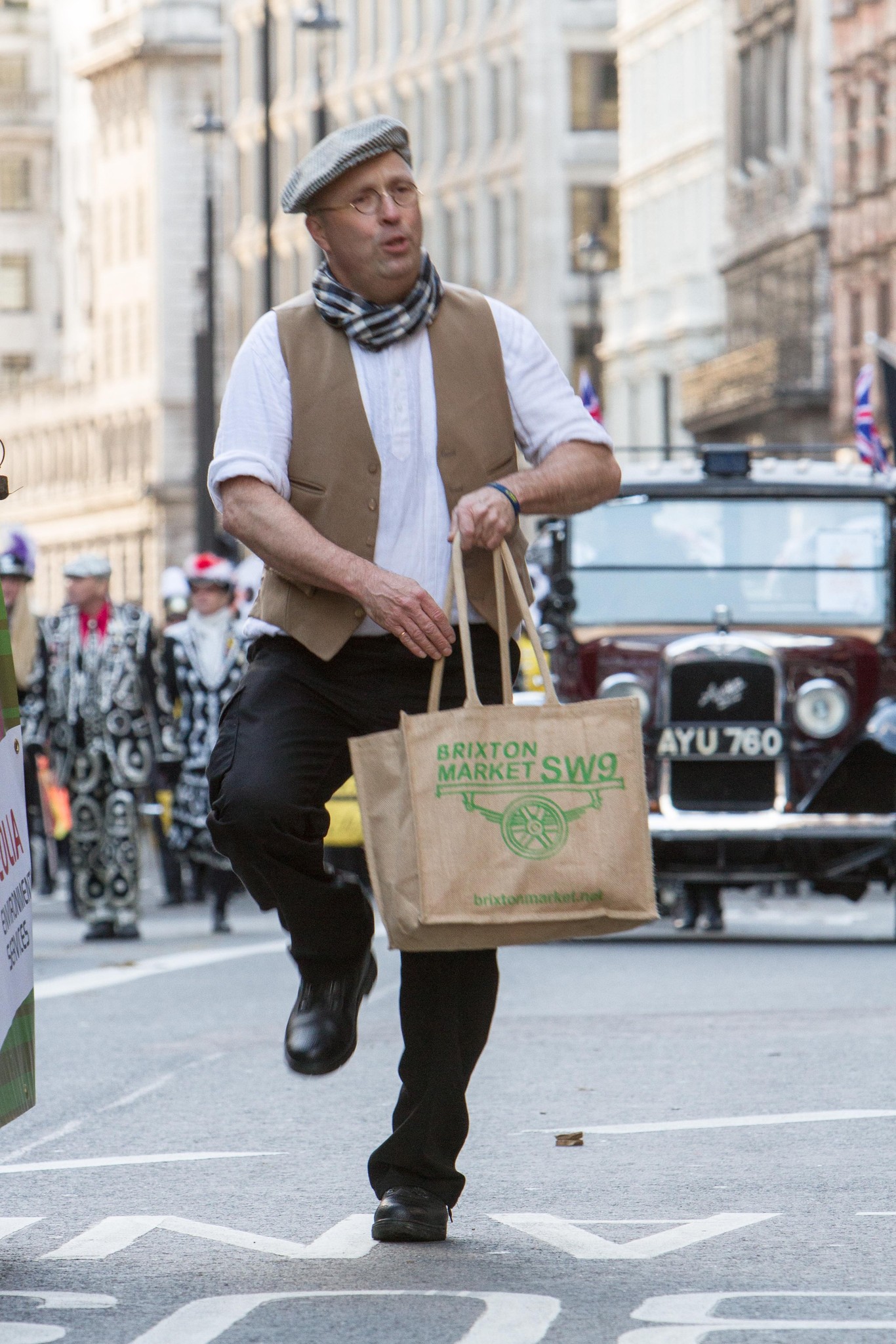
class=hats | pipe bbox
[280,115,411,213]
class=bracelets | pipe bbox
[483,481,520,518]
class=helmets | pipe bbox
[0,546,33,579]
[185,554,237,585]
[62,555,112,579]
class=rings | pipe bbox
[398,630,407,638]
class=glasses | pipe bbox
[311,180,422,216]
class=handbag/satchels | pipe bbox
[347,531,661,952]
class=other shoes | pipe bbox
[210,918,229,932]
[161,893,183,907]
[115,922,140,939]
[704,912,723,932]
[84,920,114,940]
[37,857,55,893]
[674,906,696,929]
[189,880,208,901]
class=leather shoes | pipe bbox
[371,1185,452,1243]
[285,935,377,1076]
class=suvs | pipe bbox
[545,443,896,914]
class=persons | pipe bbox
[206,115,620,1241]
[0,531,266,944]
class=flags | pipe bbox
[853,364,886,475]
[578,369,601,423]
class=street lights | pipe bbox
[571,230,608,397]
[296,5,344,262]
[188,96,228,556]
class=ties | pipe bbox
[79,619,98,720]
